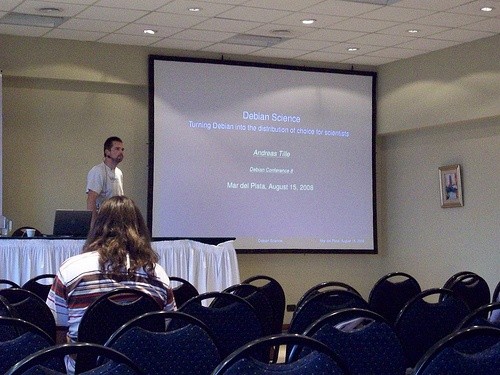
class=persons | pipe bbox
[85,137,124,230]
[46,197,176,375]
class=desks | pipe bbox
[0,237,241,308]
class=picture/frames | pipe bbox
[438,164,464,209]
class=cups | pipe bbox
[26,229,36,237]
[1,217,13,235]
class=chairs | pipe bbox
[0,270,500,375]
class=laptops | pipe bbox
[53,209,92,236]
